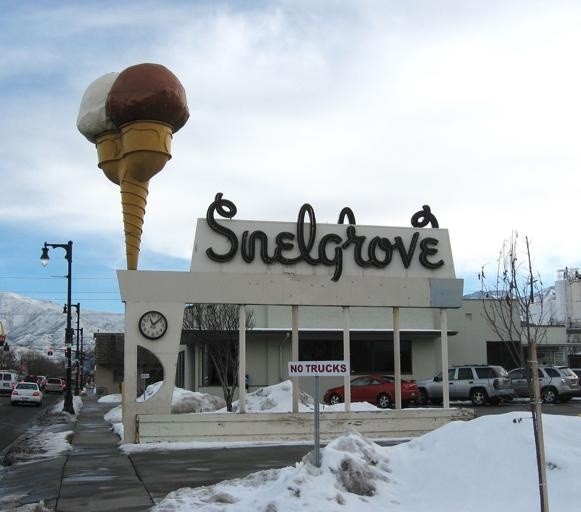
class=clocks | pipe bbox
[137,310,168,340]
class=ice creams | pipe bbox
[77,63,190,271]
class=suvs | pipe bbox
[414,364,513,406]
[508,364,579,404]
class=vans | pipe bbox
[1,370,16,394]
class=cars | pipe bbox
[12,374,47,407]
[324,374,418,407]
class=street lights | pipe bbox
[40,240,84,408]
